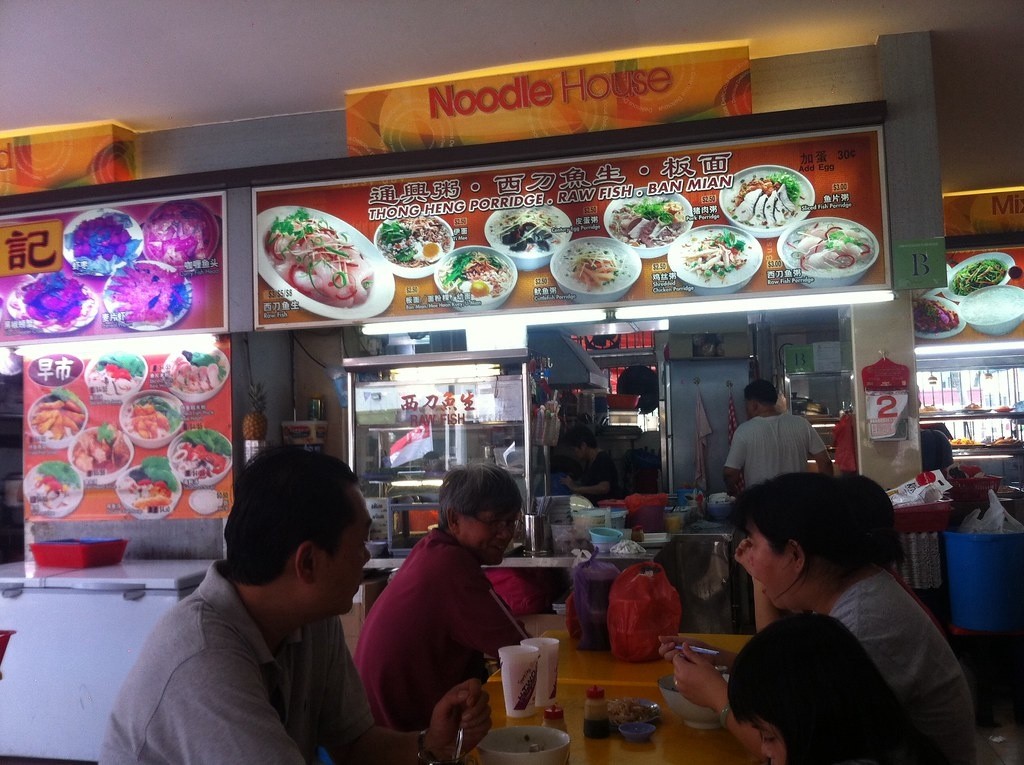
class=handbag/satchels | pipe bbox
[564,545,621,652]
[958,489,1024,534]
[605,560,682,663]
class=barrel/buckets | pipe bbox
[944,531,1024,632]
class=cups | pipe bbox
[664,513,681,533]
[417,745,466,765]
[498,645,540,718]
[520,638,559,707]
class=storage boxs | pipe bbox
[896,495,954,511]
[813,340,841,371]
[947,473,1005,502]
[280,420,329,445]
[784,343,813,373]
[894,506,958,533]
[28,535,130,569]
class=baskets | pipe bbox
[29,538,128,567]
[891,490,953,533]
[946,474,1003,501]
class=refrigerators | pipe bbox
[0,559,219,762]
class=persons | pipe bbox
[659,472,977,765]
[560,427,625,506]
[353,462,534,732]
[724,379,833,488]
[99,446,492,765]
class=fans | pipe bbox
[585,333,622,350]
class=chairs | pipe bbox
[427,680,776,765]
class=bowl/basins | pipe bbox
[549,236,642,304]
[606,394,641,409]
[776,217,879,288]
[957,285,1024,337]
[618,721,656,742]
[666,224,763,296]
[707,503,733,520]
[589,526,624,542]
[590,542,618,553]
[433,245,518,312]
[365,541,387,558]
[24,345,232,521]
[603,186,694,259]
[718,165,816,239]
[484,203,573,272]
[477,725,571,765]
[657,670,731,730]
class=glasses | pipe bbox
[472,514,524,539]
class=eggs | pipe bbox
[459,280,494,305]
[413,241,444,263]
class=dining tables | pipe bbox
[480,626,774,688]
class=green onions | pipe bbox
[564,242,624,286]
[827,231,866,250]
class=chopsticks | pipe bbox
[674,645,719,655]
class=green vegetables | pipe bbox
[692,228,746,278]
[268,209,373,297]
[25,228,191,317]
[36,352,233,491]
[768,171,800,203]
[951,258,1006,296]
[441,252,503,288]
[380,220,416,262]
[623,197,674,225]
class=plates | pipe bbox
[953,409,991,414]
[913,295,966,340]
[5,199,219,332]
[942,251,1015,302]
[258,205,396,320]
[609,697,661,726]
[373,211,455,279]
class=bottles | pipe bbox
[307,392,324,420]
[541,706,568,733]
[583,684,610,738]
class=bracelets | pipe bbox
[719,703,730,729]
[417,728,429,761]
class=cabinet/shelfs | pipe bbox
[919,411,1024,455]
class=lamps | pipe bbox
[0,345,16,369]
[357,307,610,337]
[14,330,223,364]
[927,371,938,386]
[983,369,994,380]
[953,453,1013,460]
[610,288,903,322]
